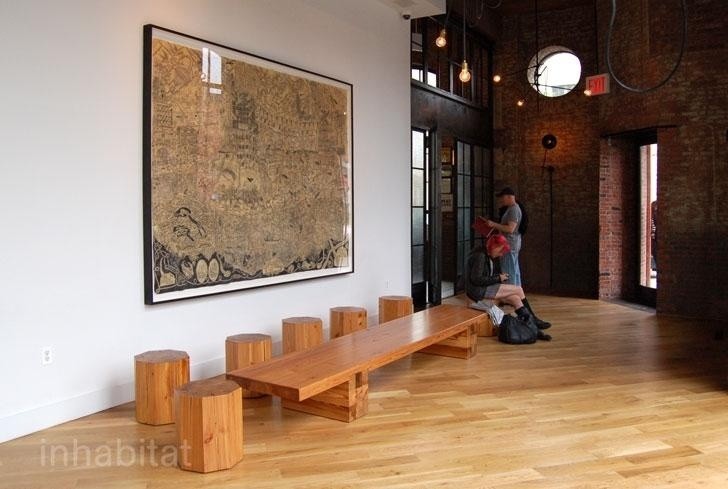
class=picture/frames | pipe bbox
[143,24,355,306]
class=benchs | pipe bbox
[227,301,502,424]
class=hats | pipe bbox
[495,187,514,196]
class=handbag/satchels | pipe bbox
[499,313,538,344]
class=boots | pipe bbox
[515,306,552,341]
[522,296,551,329]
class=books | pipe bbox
[470,216,495,238]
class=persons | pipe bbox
[463,234,552,342]
[486,188,523,287]
